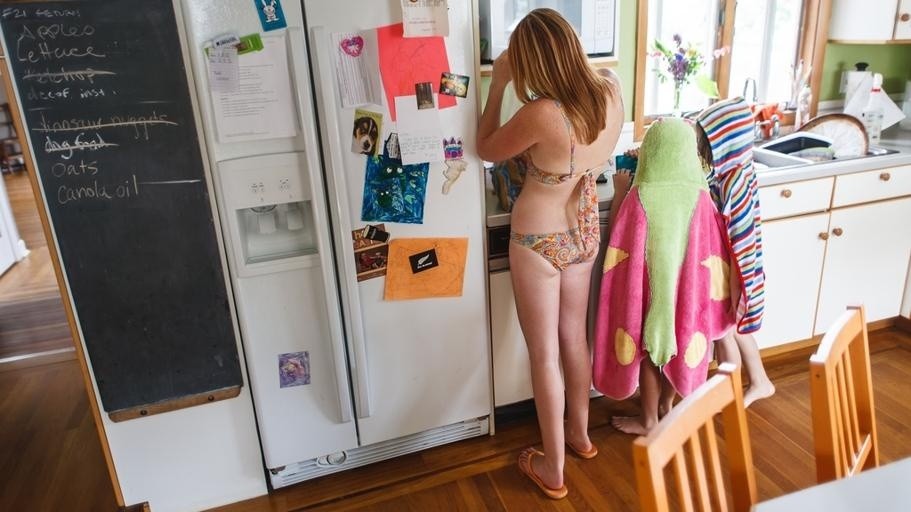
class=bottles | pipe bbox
[860,72,884,151]
[793,84,813,132]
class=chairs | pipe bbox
[632,363,758,512]
[810,303,882,483]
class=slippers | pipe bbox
[566,437,598,459]
[519,450,568,499]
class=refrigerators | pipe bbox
[177,1,493,491]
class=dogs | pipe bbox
[351,117,378,157]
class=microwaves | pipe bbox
[479,0,615,61]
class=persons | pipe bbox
[474,8,625,501]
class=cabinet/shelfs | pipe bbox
[828,0,911,45]
[752,164,911,364]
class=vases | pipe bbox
[646,32,733,109]
[670,79,688,115]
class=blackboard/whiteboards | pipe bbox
[2,3,246,417]
